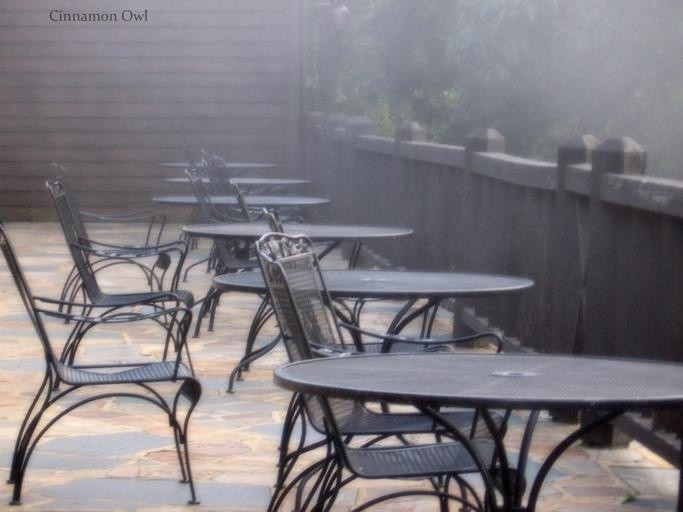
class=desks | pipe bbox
[149,159,683,511]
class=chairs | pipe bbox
[45,180,196,390]
[1,224,209,506]
[52,177,165,313]
